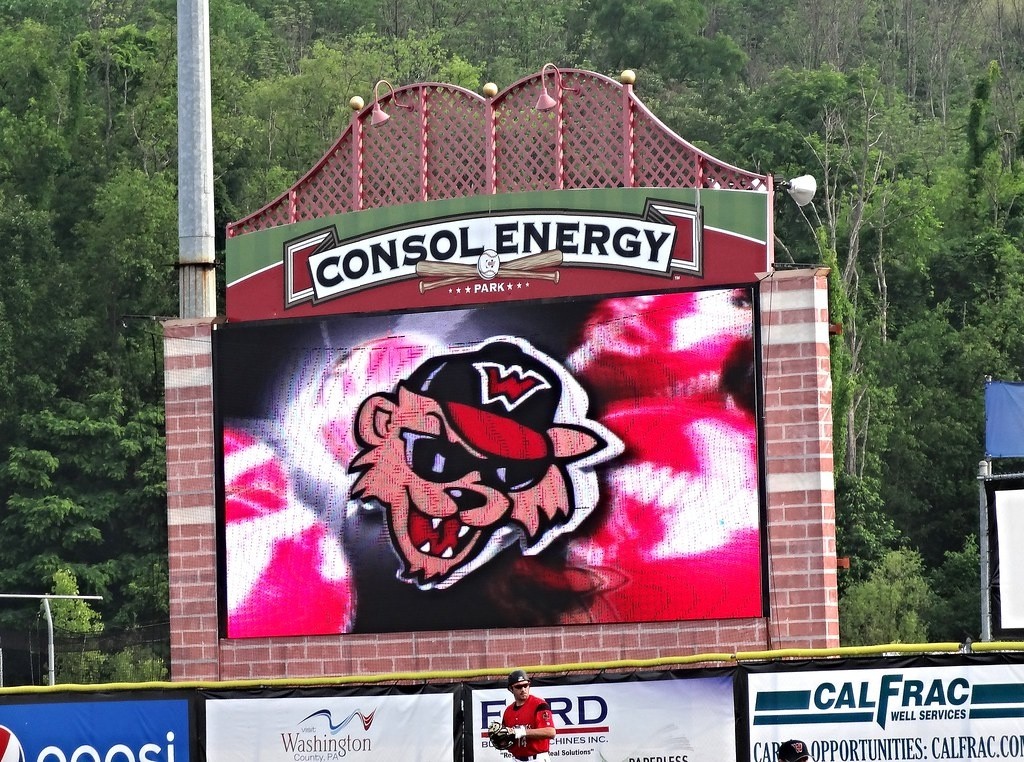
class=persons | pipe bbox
[777,740,811,762]
[488,669,556,762]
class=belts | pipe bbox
[515,755,536,761]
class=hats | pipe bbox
[778,740,813,762]
[507,670,531,687]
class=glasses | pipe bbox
[514,684,528,690]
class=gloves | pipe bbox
[506,725,526,739]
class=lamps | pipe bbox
[774,173,817,207]
[370,79,415,129]
[535,63,580,113]
